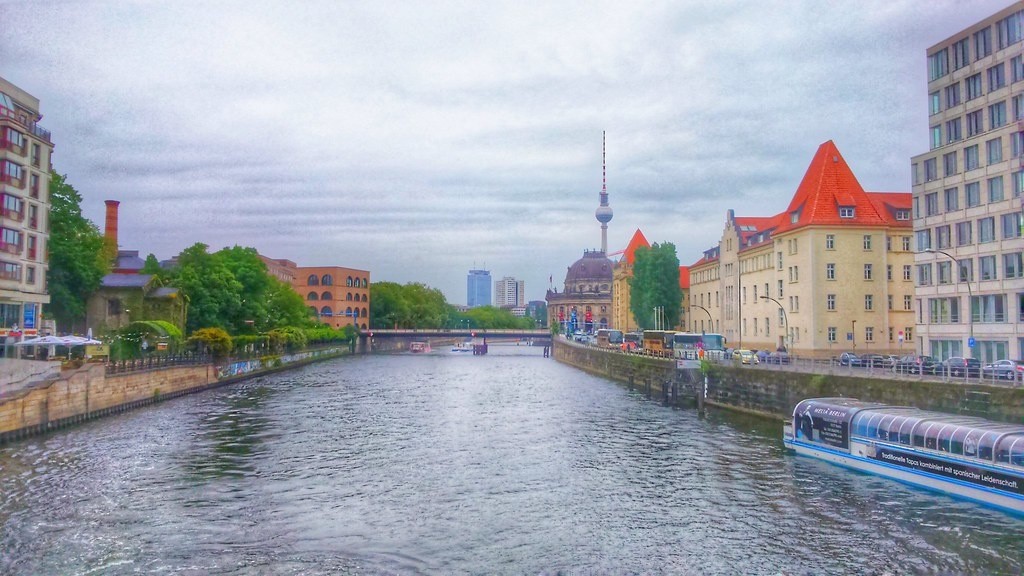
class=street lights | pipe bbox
[759,295,789,348]
[689,304,715,334]
[924,248,974,355]
[851,319,857,355]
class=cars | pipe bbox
[756,351,770,362]
[765,350,789,364]
[725,349,734,358]
[977,360,1024,381]
[891,355,939,375]
[732,348,759,364]
[566,329,598,343]
[840,353,900,369]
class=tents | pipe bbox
[15,334,102,361]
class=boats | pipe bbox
[780,392,1024,523]
[408,340,432,353]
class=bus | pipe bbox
[597,329,624,349]
[622,332,644,354]
[672,332,728,363]
[642,330,683,355]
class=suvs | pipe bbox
[929,357,981,377]
